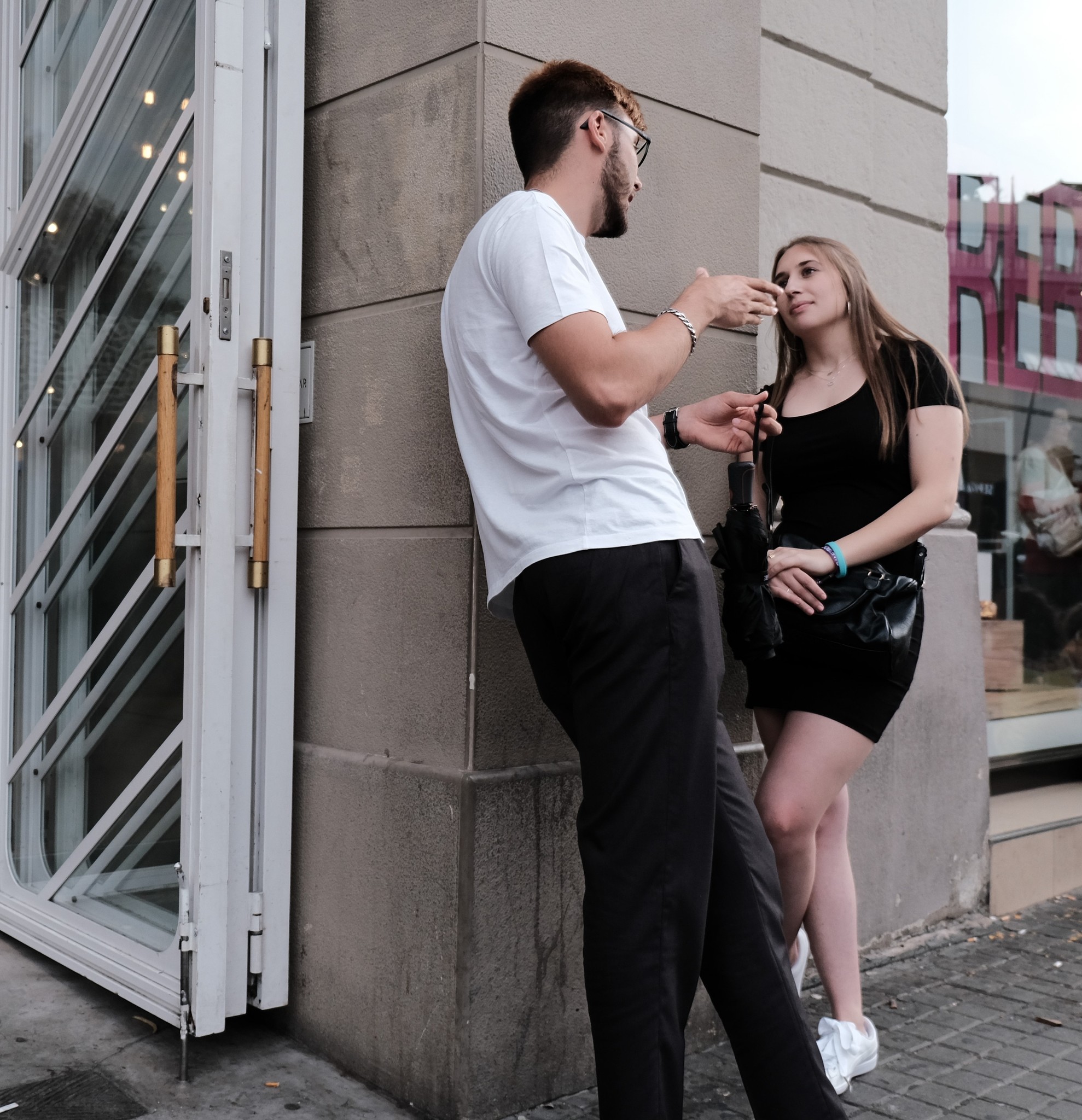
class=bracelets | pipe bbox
[657,308,696,355]
[821,541,846,578]
[663,407,691,451]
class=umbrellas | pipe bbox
[711,402,782,662]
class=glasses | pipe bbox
[580,109,652,169]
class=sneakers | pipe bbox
[790,928,809,998]
[818,1015,878,1095]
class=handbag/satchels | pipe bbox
[1032,502,1082,557]
[775,560,926,658]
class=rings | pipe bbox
[787,588,790,592]
[768,554,774,559]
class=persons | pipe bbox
[743,237,973,1088]
[1011,395,1082,674]
[437,62,847,1120]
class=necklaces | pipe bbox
[804,346,861,388]
